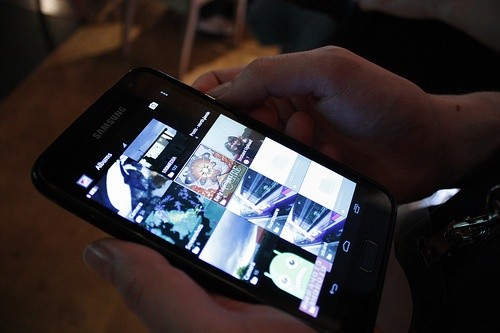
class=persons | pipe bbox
[83,45,500,333]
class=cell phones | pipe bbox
[30,66,398,333]
